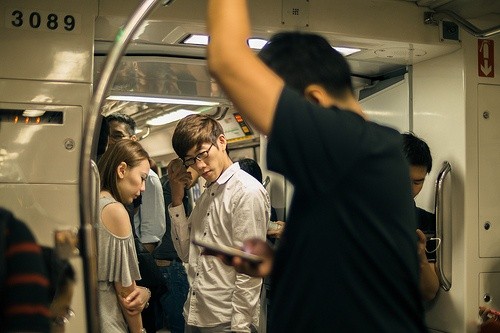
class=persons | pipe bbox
[1,113,500,333]
[206,0,427,333]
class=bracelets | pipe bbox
[171,203,181,207]
[140,286,151,308]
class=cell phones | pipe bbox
[193,239,263,264]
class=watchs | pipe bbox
[140,328,147,333]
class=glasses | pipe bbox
[109,135,132,139]
[183,137,216,167]
[54,307,76,329]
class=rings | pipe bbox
[128,305,134,311]
